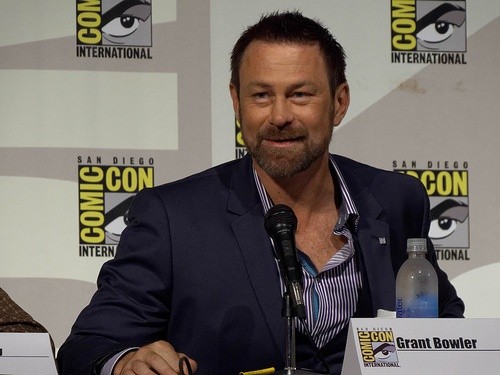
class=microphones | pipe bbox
[264,203,307,321]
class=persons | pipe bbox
[57,10,466,375]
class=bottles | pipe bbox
[395,238,439,318]
